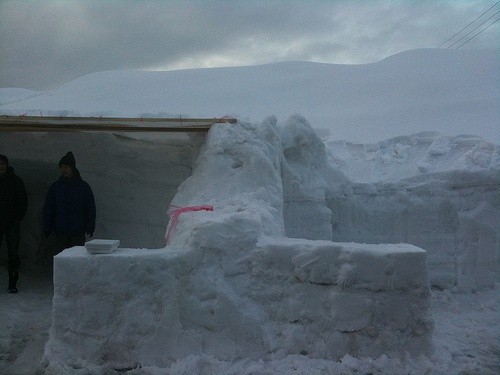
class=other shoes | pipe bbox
[8,285,16,292]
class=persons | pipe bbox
[43,151,97,278]
[0,155,28,293]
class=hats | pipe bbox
[59,151,76,168]
